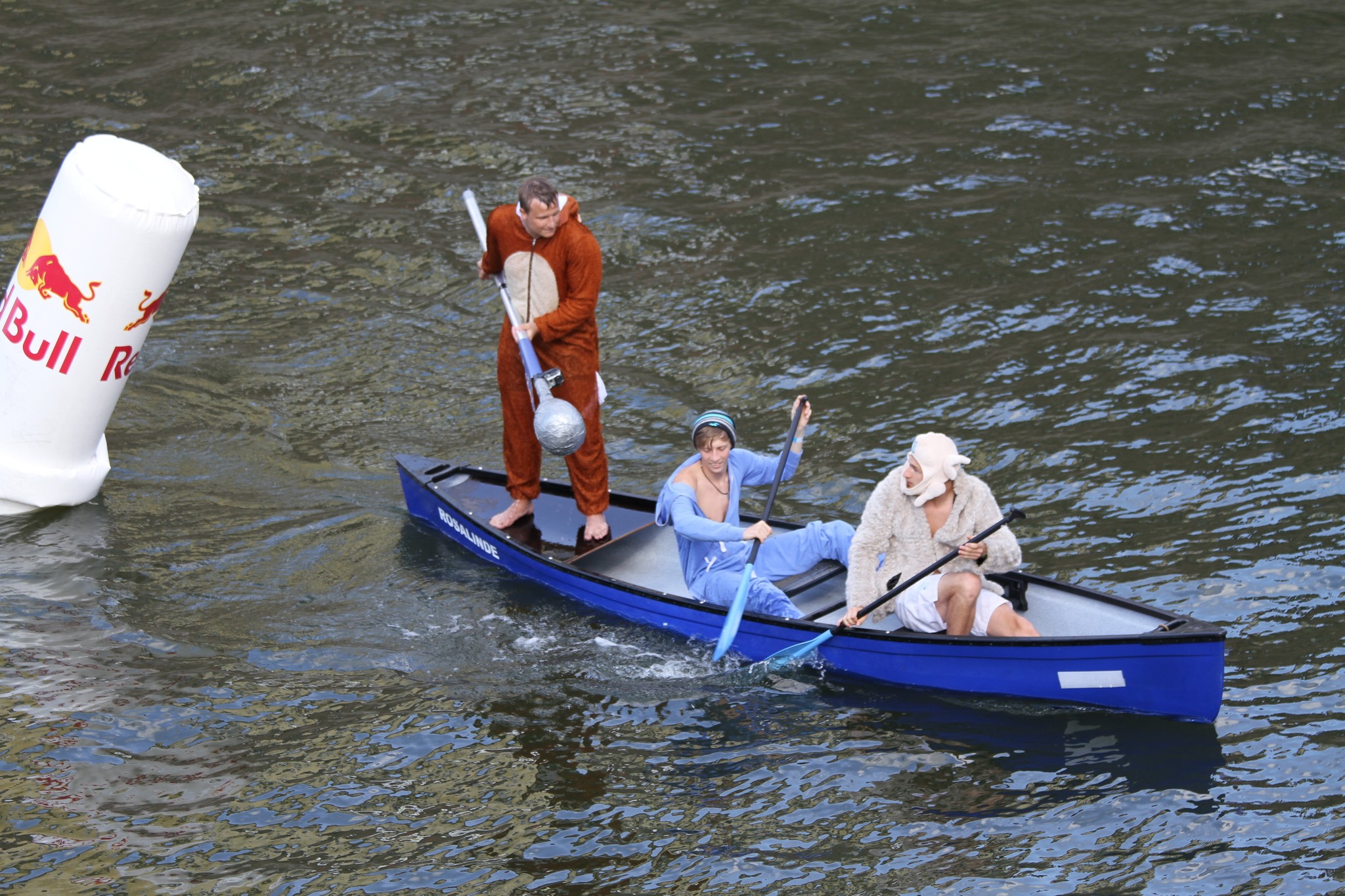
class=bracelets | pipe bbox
[793,437,805,443]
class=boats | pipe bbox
[392,452,1227,724]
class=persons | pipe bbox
[655,394,886,621]
[478,177,610,539]
[831,429,1041,637]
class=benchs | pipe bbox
[773,561,852,598]
[895,583,1021,635]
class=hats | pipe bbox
[900,431,971,504]
[693,410,738,452]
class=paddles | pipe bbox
[711,397,808,663]
[762,506,1027,668]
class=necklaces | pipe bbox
[700,458,730,495]
